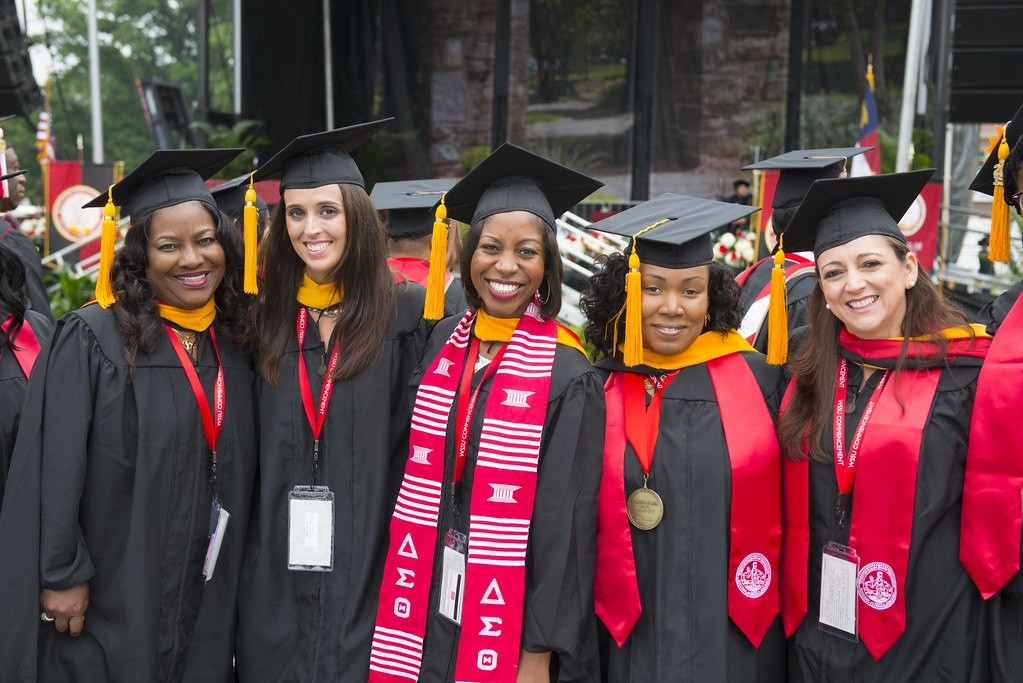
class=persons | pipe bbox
[1,104,1023,683]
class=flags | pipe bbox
[850,72,882,176]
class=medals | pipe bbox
[627,487,664,530]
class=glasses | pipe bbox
[1005,190,1023,215]
[812,254,885,287]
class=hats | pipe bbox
[368,178,458,236]
[0,114,16,199]
[740,146,875,209]
[766,168,937,366]
[967,103,1023,262]
[240,117,396,295]
[82,147,246,309]
[422,142,606,321]
[585,192,762,368]
[208,172,268,219]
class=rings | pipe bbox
[41,613,54,622]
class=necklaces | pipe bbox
[307,307,345,318]
[171,327,198,350]
